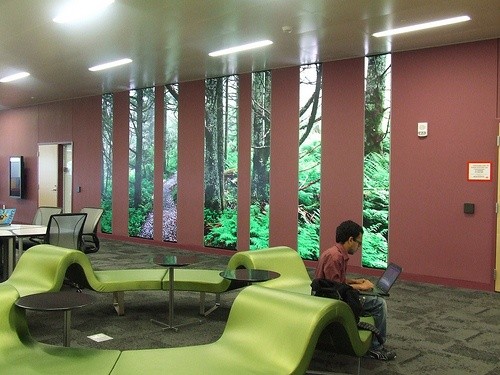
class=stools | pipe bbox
[219,269,281,288]
[150,255,203,334]
[22,293,97,348]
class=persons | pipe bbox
[314,220,397,361]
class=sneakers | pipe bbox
[364,347,397,361]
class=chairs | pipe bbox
[14,205,63,251]
[75,207,105,254]
[45,213,88,250]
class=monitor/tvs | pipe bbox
[9,156,24,199]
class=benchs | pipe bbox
[0,244,375,375]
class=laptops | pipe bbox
[358,262,402,296]
[0,209,16,226]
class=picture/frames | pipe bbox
[7,155,23,199]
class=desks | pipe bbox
[0,222,47,279]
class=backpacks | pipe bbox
[310,278,363,324]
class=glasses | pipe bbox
[353,238,362,247]
